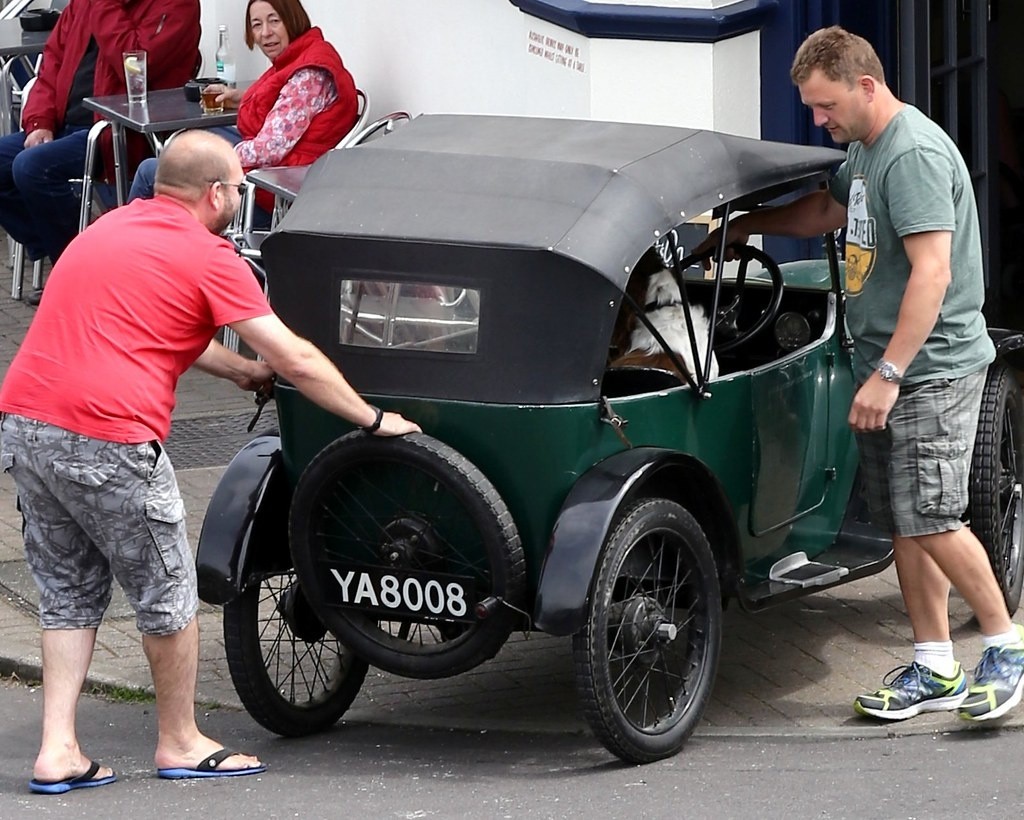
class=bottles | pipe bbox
[216,24,237,89]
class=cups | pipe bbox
[199,84,224,113]
[122,49,147,103]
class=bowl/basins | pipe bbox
[19,8,61,32]
[184,77,227,102]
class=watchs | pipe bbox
[877,358,903,384]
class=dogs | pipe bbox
[628,266,719,386]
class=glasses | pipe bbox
[208,177,248,195]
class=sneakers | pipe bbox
[852,660,969,720]
[958,624,1024,722]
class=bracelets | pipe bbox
[363,404,383,432]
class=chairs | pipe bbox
[603,363,682,398]
[0,0,478,404]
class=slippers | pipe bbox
[157,746,268,779]
[30,759,117,794]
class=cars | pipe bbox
[192,112,1024,765]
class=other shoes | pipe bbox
[28,287,44,304]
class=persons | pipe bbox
[691,27,1024,720]
[0,129,424,793]
[127,0,358,228]
[0,1,202,269]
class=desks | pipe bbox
[0,18,53,138]
[79,78,259,233]
[247,166,310,224]
